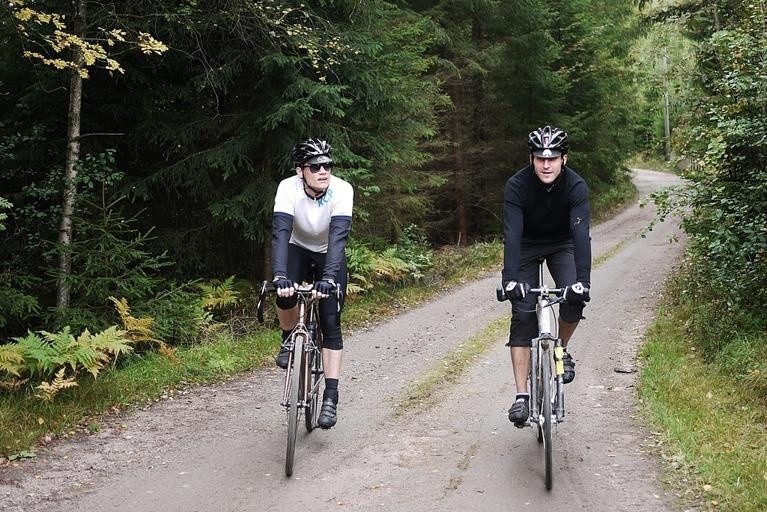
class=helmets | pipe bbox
[290,139,333,163]
[528,126,569,158]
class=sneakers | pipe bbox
[318,389,338,426]
[563,351,575,384]
[276,344,294,368]
[508,397,529,422]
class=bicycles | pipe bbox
[256,264,336,477]
[495,257,591,491]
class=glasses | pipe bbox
[302,164,329,173]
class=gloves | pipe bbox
[505,280,530,303]
[566,281,590,304]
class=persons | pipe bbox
[501,123,594,428]
[270,137,355,429]
